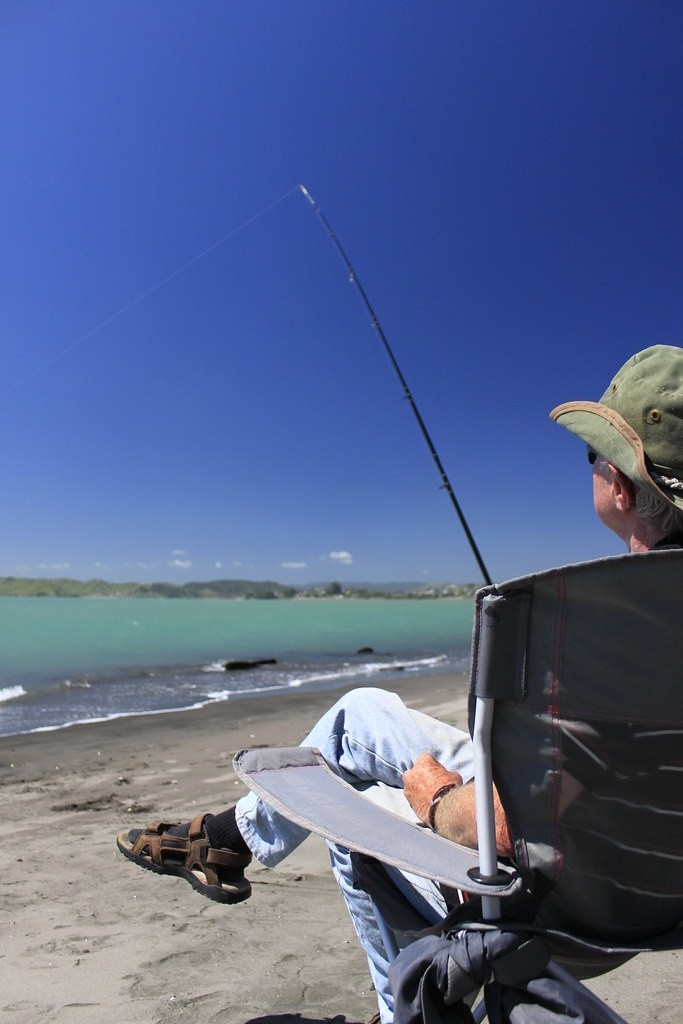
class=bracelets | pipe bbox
[428,779,456,830]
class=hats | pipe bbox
[548,342,683,516]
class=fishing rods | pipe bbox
[297,182,492,585]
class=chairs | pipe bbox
[232,546,683,1024]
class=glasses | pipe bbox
[586,443,597,465]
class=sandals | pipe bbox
[116,813,253,905]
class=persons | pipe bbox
[116,342,683,1024]
